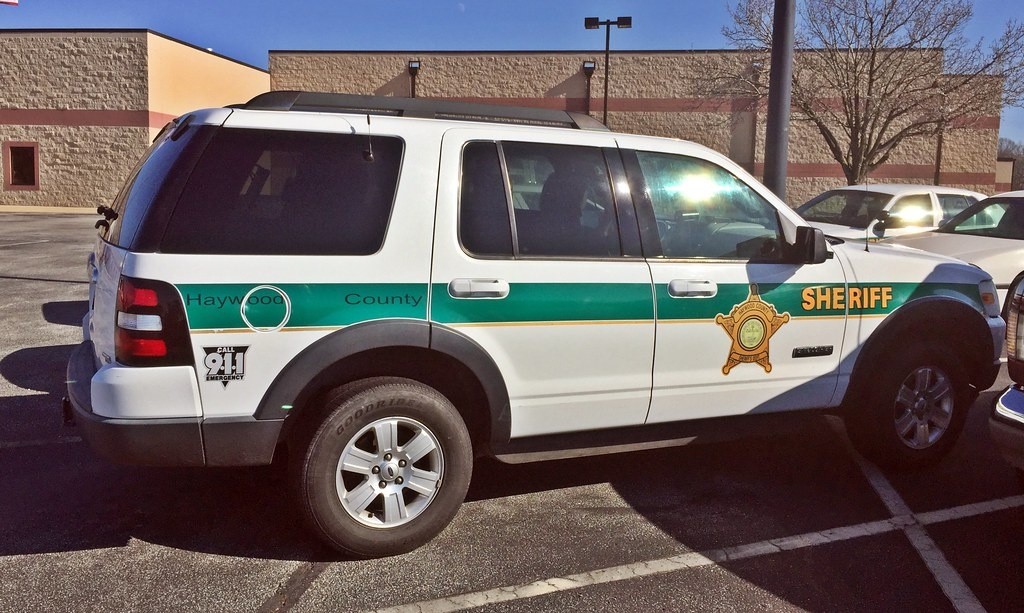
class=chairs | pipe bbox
[463,172,515,256]
[525,170,590,256]
[851,200,882,228]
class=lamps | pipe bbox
[583,61,596,76]
[409,61,420,75]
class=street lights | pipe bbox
[582,60,596,116]
[585,17,632,126]
[407,60,421,97]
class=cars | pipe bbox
[795,185,1006,245]
[875,190,1024,337]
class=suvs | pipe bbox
[60,89,1008,558]
